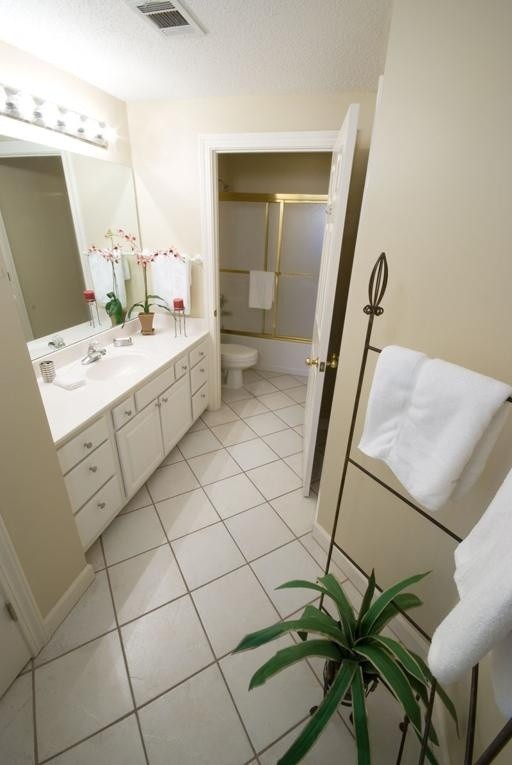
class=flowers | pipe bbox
[116,227,183,321]
[87,229,126,326]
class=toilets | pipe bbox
[220,343,258,390]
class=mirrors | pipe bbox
[1,135,153,361]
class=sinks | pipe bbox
[78,353,147,379]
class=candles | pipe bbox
[174,297,183,309]
[83,290,95,302]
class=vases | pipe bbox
[110,309,127,328]
[138,311,156,335]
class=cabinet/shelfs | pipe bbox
[56,341,210,554]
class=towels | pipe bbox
[88,248,131,309]
[53,374,89,390]
[151,252,192,315]
[249,270,275,310]
[357,346,511,512]
[428,467,511,720]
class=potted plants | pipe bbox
[232,566,462,765]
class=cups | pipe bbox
[38,360,57,385]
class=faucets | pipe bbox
[80,340,107,365]
[47,334,66,349]
